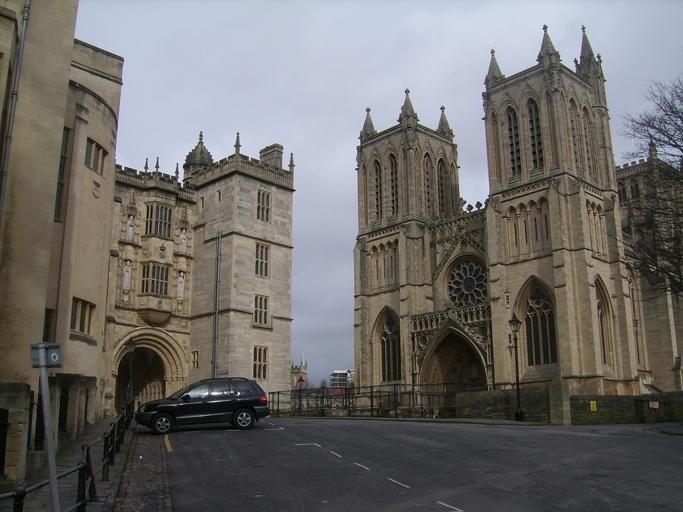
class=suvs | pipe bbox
[135,376,271,434]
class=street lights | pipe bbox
[507,312,523,422]
[296,376,304,417]
[124,335,137,420]
